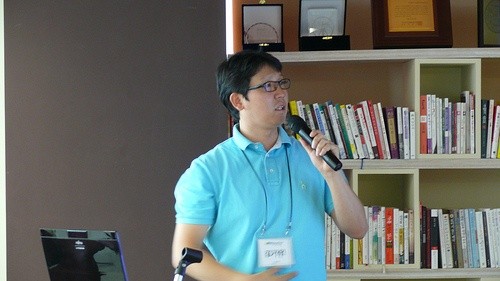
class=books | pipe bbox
[420,90,500,159]
[325,205,414,270]
[419,203,500,269]
[286,100,416,160]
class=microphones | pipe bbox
[288,115,342,171]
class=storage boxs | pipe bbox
[297,0,351,50]
[242,4,286,52]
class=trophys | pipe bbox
[246,22,278,43]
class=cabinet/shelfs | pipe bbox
[222,0,500,281]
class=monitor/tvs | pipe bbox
[40,228,129,281]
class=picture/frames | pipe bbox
[476,0,500,48]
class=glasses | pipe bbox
[247,78,291,92]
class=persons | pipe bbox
[172,52,368,281]
